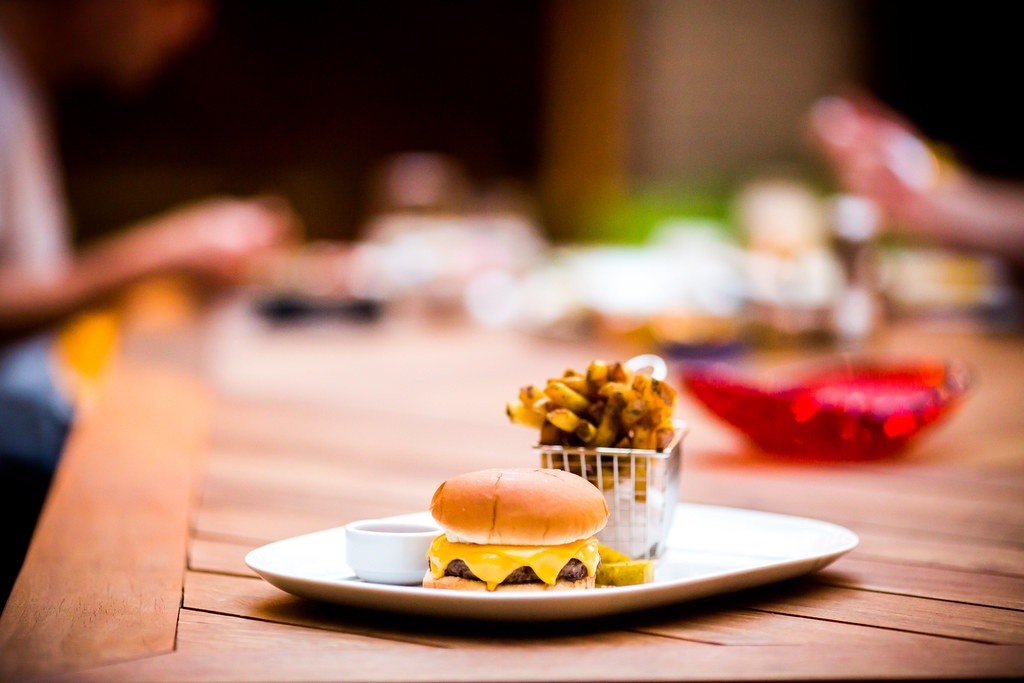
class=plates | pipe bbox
[244,501,860,624]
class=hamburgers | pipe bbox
[421,467,609,593]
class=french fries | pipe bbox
[506,358,677,500]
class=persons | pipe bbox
[810,99,1023,255]
[0,1,297,613]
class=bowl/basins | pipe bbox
[346,521,443,586]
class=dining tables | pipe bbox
[0,315,1024,683]
